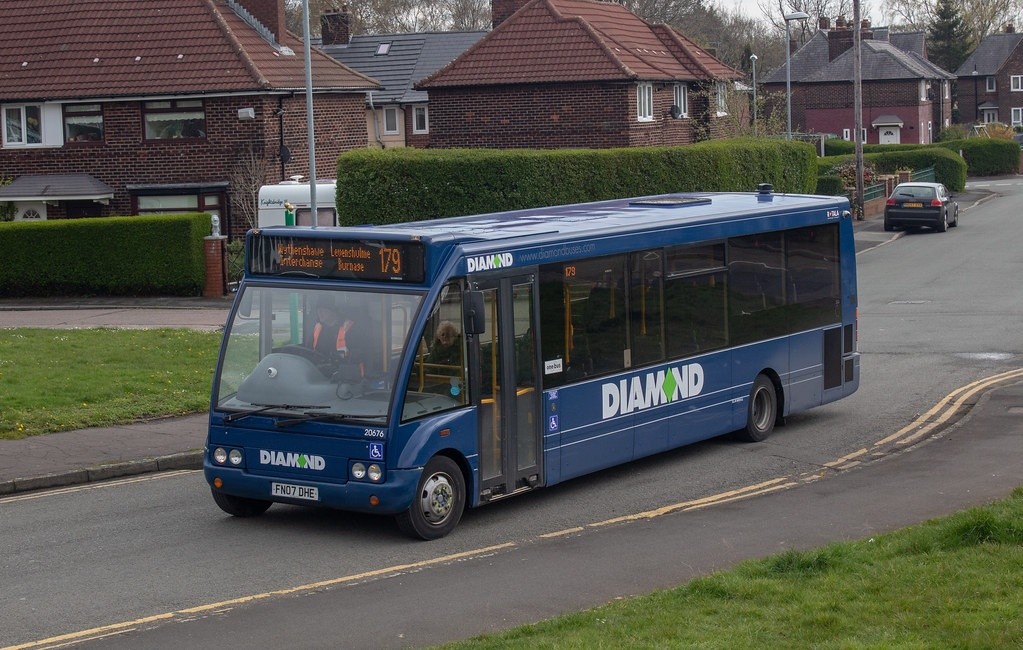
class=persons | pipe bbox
[312,294,358,365]
[428,321,461,376]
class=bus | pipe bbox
[202,184,860,540]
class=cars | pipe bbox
[884,182,958,232]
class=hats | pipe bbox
[316,295,335,309]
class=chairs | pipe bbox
[535,281,821,372]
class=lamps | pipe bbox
[237,108,255,121]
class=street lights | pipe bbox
[750,54,758,119]
[784,12,809,140]
